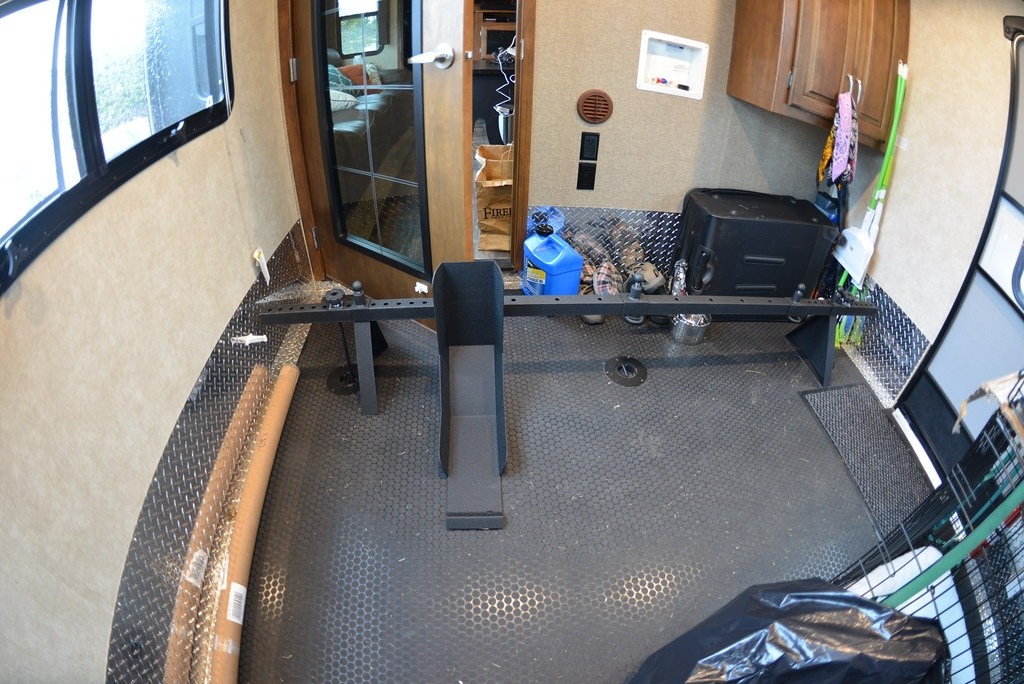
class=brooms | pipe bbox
[829,57,904,351]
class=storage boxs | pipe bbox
[663,187,839,323]
[474,144,512,252]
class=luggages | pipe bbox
[671,187,848,321]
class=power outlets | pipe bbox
[575,162,597,190]
[579,131,600,161]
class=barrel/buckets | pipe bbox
[666,310,712,347]
[526,206,566,242]
[522,222,584,297]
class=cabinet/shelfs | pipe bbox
[726,0,911,153]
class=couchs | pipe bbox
[327,47,412,209]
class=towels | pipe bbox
[815,93,859,191]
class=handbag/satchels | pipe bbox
[474,140,515,252]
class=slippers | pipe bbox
[644,284,674,324]
[578,284,606,324]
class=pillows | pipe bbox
[328,63,359,95]
[329,88,359,112]
[364,63,388,93]
[337,65,378,93]
